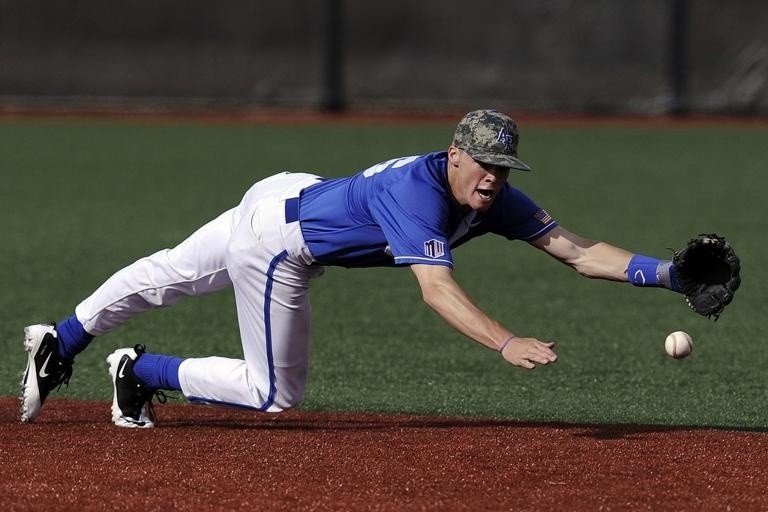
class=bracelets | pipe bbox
[499,333,518,354]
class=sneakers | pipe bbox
[20,324,74,423]
[106,343,156,429]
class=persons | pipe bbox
[18,108,743,430]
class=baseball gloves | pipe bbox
[666,233,741,320]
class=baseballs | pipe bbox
[666,330,692,358]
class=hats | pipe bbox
[452,108,532,172]
